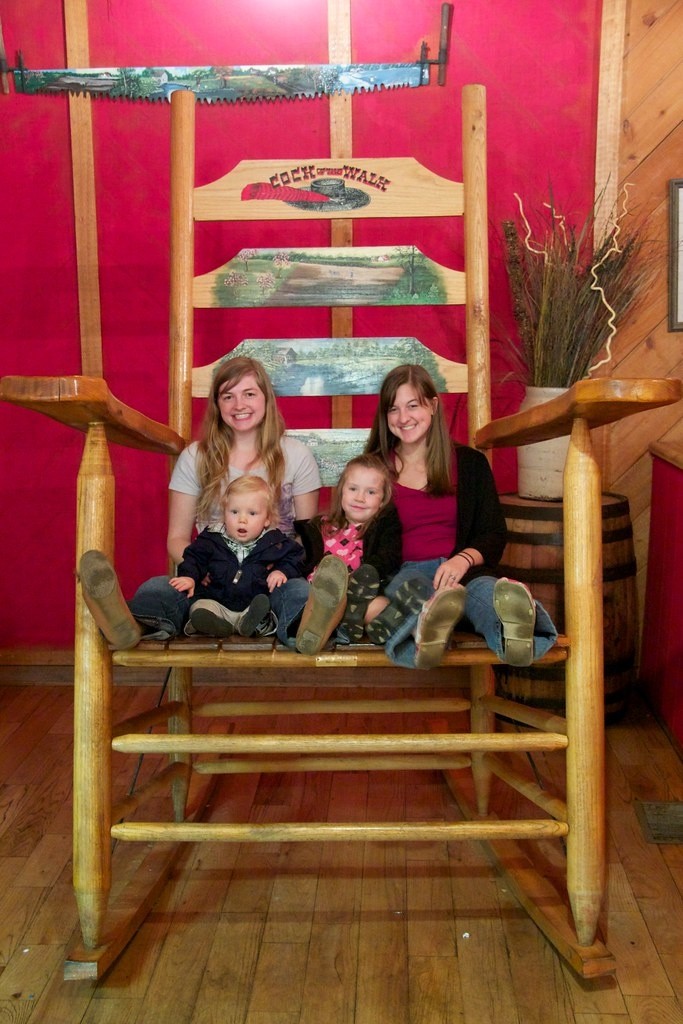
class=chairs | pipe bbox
[0,84,683,982]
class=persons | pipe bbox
[365,364,558,671]
[79,357,348,655]
[169,477,307,639]
[292,454,429,645]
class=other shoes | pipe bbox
[190,607,234,639]
[493,577,537,667]
[339,564,379,643]
[414,584,467,670]
[79,550,141,651]
[295,555,349,656]
[239,594,271,637]
[366,576,436,645]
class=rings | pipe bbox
[449,575,456,580]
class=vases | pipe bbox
[515,385,570,501]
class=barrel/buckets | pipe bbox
[498,491,637,726]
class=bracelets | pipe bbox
[456,551,475,567]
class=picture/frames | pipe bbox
[667,177,683,332]
